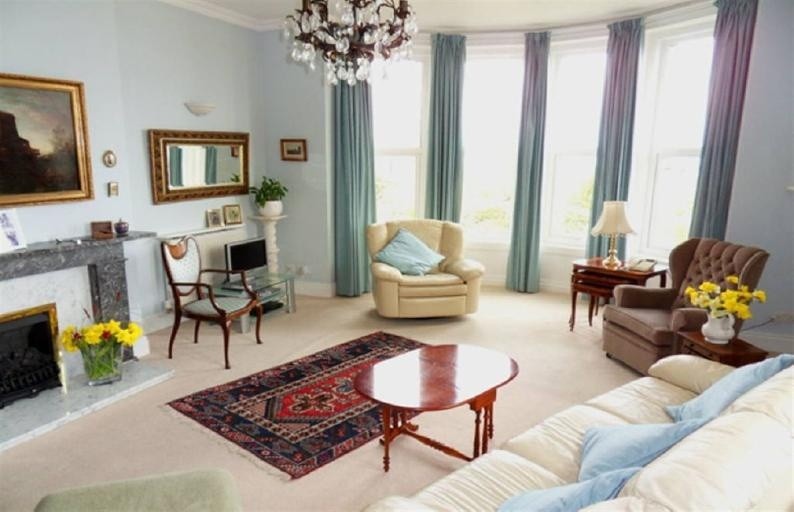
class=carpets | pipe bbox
[169,331,429,480]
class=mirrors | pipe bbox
[149,130,251,205]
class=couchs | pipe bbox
[365,219,485,318]
[366,354,794,512]
[34,469,243,512]
[602,239,769,376]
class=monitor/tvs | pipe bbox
[224,238,268,282]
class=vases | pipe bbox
[78,341,124,385]
[700,313,736,344]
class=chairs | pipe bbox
[161,237,262,368]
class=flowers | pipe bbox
[61,319,141,351]
[685,276,766,318]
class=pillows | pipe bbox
[498,468,641,512]
[666,354,794,423]
[577,417,714,483]
[374,230,445,275]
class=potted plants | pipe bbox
[250,176,288,217]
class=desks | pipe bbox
[569,257,667,331]
[248,214,287,287]
[678,331,768,367]
[204,272,296,334]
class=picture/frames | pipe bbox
[230,147,239,157]
[207,204,242,227]
[0,72,95,209]
[281,138,307,161]
[0,208,27,252]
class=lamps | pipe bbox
[592,201,635,269]
[283,0,419,85]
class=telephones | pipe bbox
[628,257,657,272]
